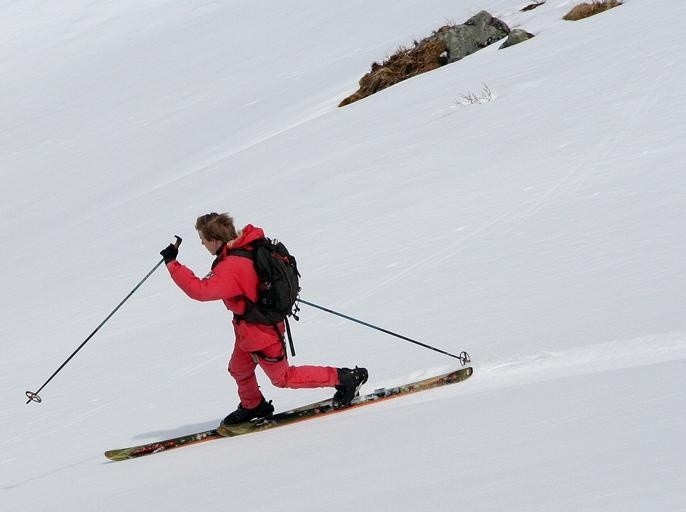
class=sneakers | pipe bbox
[334,368,368,403]
[223,396,265,425]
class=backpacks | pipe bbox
[211,238,301,326]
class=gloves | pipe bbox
[160,244,178,265]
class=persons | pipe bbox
[159,212,369,429]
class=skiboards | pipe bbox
[104,367,472,462]
